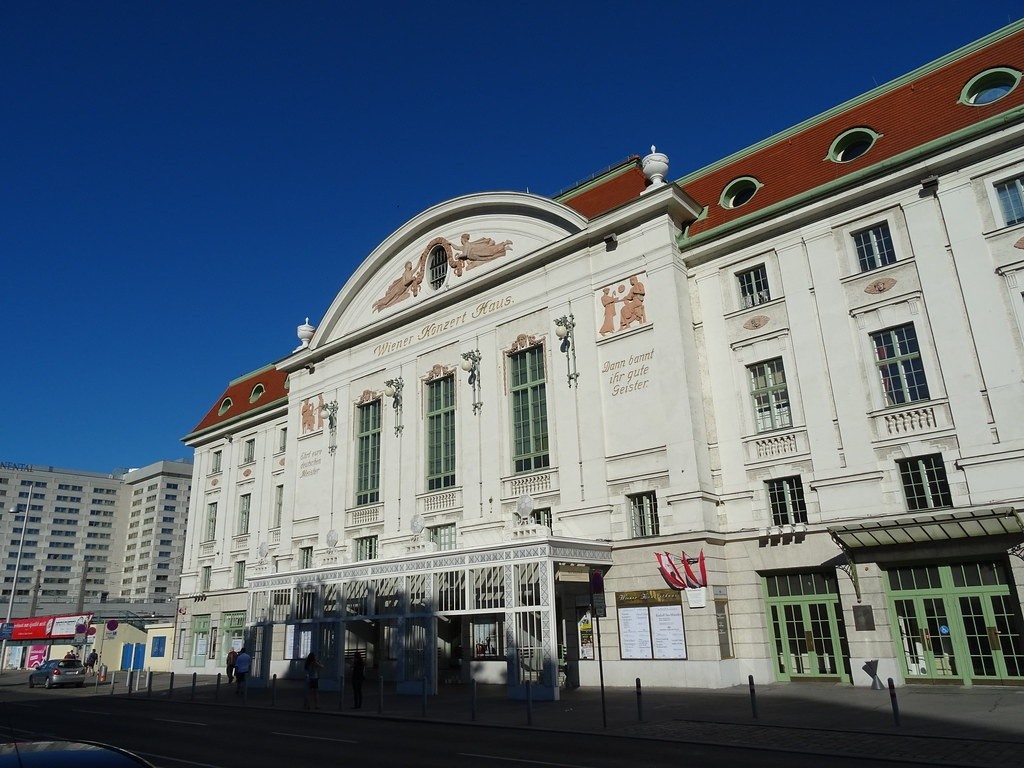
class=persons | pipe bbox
[86,649,98,677]
[371,260,422,314]
[505,333,546,354]
[301,394,324,435]
[355,390,382,405]
[599,276,645,337]
[424,364,456,381]
[874,280,886,292]
[226,647,251,695]
[751,318,760,328]
[447,234,513,271]
[64,650,75,660]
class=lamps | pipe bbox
[320,400,337,454]
[553,314,581,386]
[460,349,483,416]
[383,377,404,437]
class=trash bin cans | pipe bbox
[99,664,107,682]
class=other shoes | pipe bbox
[308,705,312,709]
[350,706,360,709]
[229,676,234,684]
[315,704,320,709]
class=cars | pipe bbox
[29,659,86,689]
[0,739,155,768]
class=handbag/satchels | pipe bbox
[362,665,366,679]
